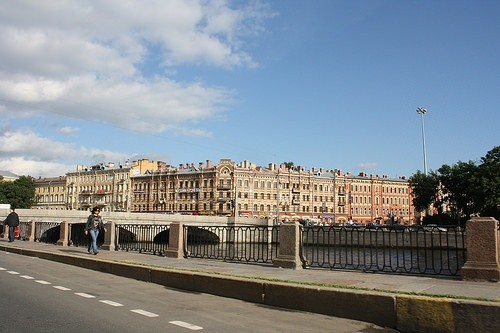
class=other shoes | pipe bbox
[94,251,99,255]
[87,249,93,254]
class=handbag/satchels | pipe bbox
[12,226,20,237]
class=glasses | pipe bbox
[94,210,100,212]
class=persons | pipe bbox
[2,207,20,242]
[84,206,107,255]
